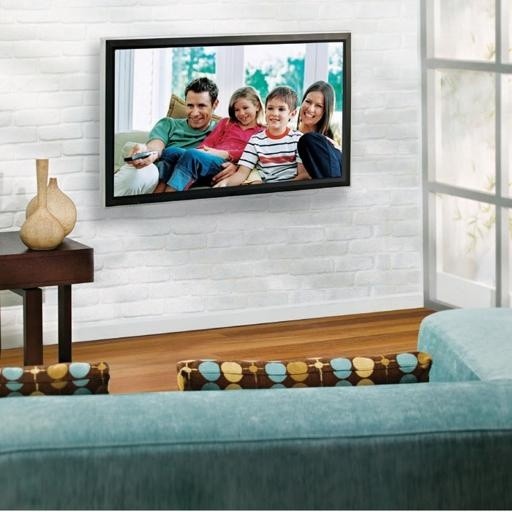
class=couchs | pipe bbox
[0,305,512,510]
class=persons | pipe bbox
[296,80,342,179]
[152,86,264,193]
[212,86,313,188]
[114,77,221,196]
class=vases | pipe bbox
[25,178,78,238]
[20,160,64,251]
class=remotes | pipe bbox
[122,150,155,161]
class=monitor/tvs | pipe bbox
[102,33,351,209]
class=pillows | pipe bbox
[177,351,432,391]
[0,361,110,397]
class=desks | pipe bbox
[0,230,94,366]
